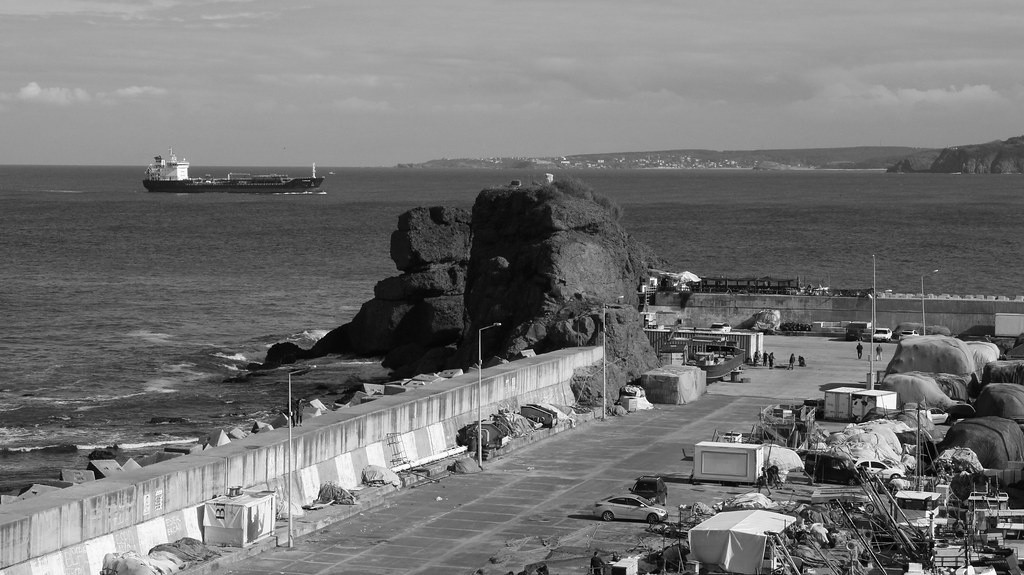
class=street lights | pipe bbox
[921,269,939,335]
[479,321,501,473]
[601,295,625,422]
[868,293,873,390]
[288,365,316,549]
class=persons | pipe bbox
[287,397,296,427]
[758,467,771,496]
[590,551,605,575]
[787,353,795,370]
[769,352,774,369]
[856,343,863,359]
[763,351,768,365]
[876,344,883,361]
[296,399,304,426]
[798,356,806,367]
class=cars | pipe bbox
[709,321,732,333]
[845,327,863,342]
[898,329,919,342]
[873,327,892,343]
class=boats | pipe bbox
[686,346,745,379]
[143,147,325,195]
[656,271,874,297]
